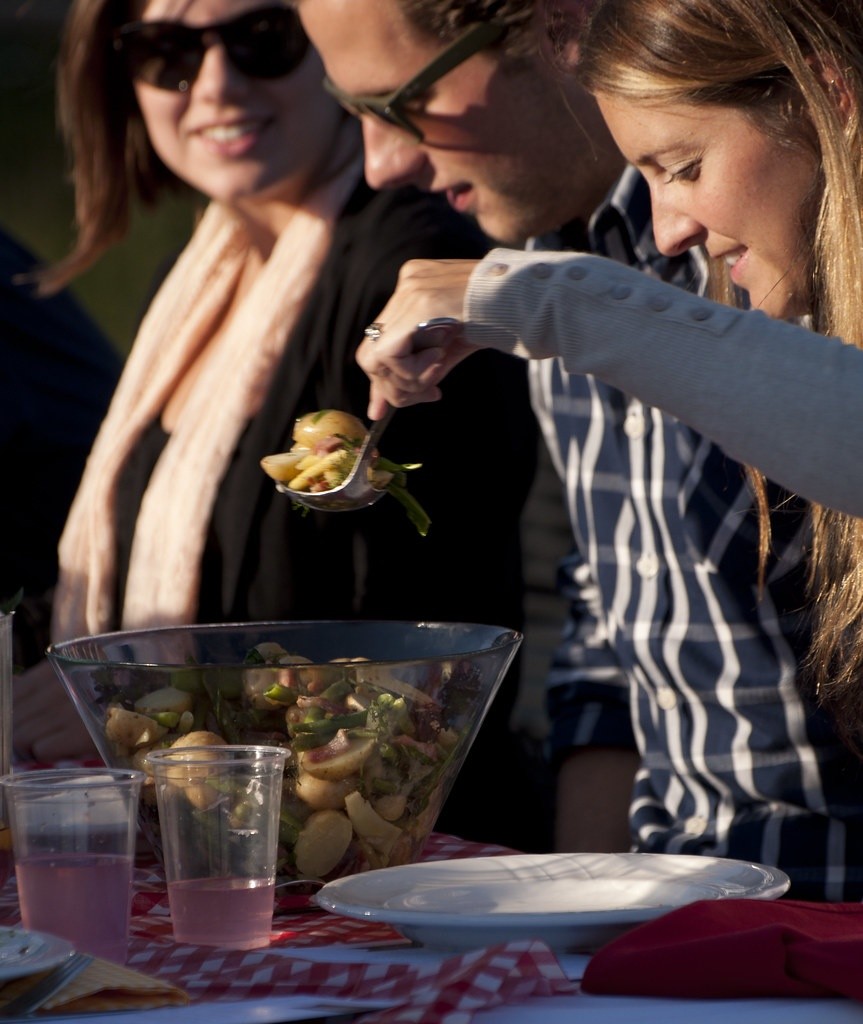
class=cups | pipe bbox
[0,768,147,967]
[145,745,292,950]
[0,610,17,851]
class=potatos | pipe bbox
[260,412,368,490]
[103,641,460,882]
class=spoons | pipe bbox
[274,318,460,513]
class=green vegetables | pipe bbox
[380,456,431,537]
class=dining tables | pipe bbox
[2,737,863,1024]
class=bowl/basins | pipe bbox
[46,619,523,895]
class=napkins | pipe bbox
[581,871,863,1007]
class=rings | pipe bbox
[364,323,384,340]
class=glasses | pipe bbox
[116,4,309,91]
[321,20,507,144]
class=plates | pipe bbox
[0,926,74,981]
[316,853,790,951]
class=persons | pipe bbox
[31,1,862,907]
[353,0,863,721]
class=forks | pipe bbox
[0,951,93,1019]
